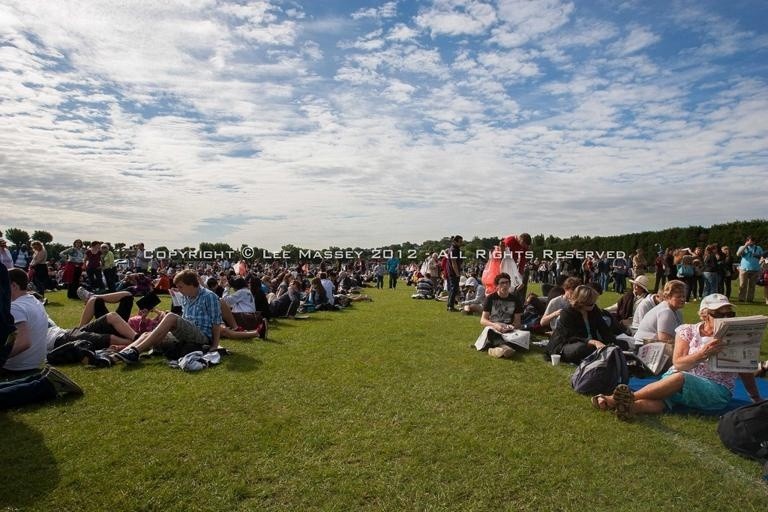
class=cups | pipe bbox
[550,354,561,366]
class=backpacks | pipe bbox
[571,344,629,394]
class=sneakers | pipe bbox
[256,318,268,340]
[115,346,140,365]
[613,384,634,421]
[488,345,517,358]
[77,286,94,304]
[84,350,113,368]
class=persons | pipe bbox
[1,232,403,410]
[405,231,767,460]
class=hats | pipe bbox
[628,275,650,293]
[699,293,737,311]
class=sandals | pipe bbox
[590,394,614,411]
